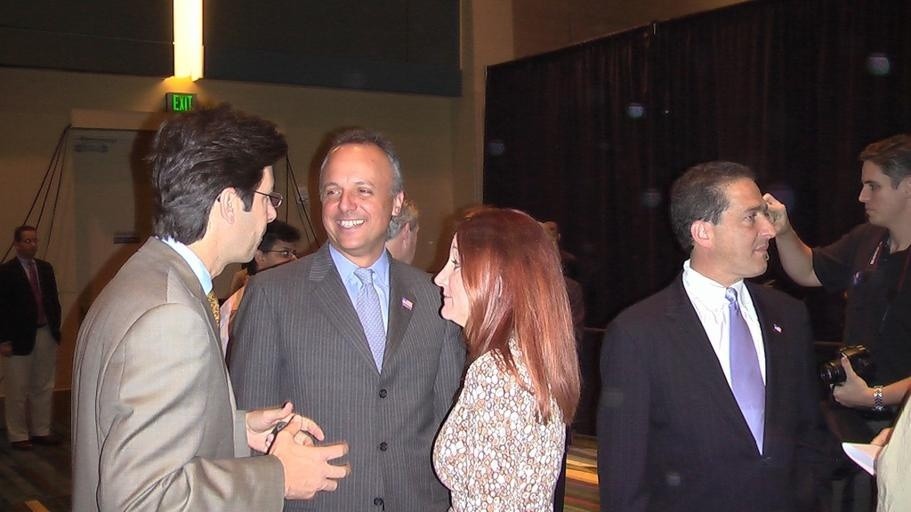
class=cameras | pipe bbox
[820,343,874,383]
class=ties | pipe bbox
[725,287,765,455]
[354,267,386,374]
[29,262,44,325]
[206,288,220,333]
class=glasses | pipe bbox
[218,184,283,207]
[266,248,297,258]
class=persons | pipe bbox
[0,225,62,449]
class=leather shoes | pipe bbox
[15,440,32,449]
[37,436,56,445]
